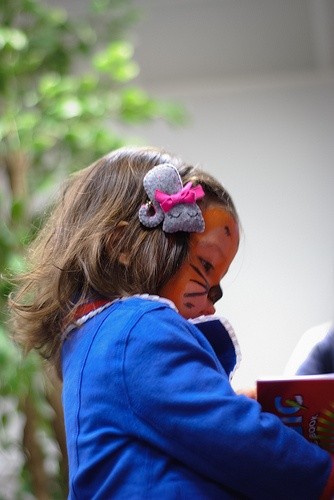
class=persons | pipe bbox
[5,144,333,499]
